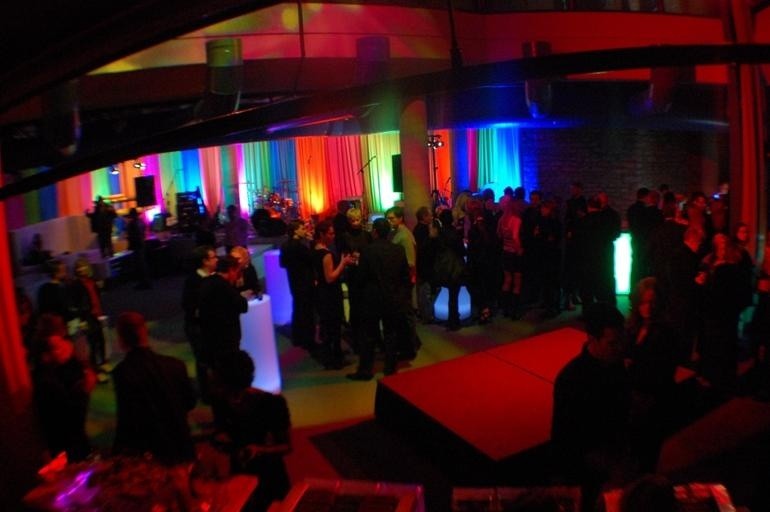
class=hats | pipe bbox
[123,208,139,217]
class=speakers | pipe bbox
[135,175,155,207]
[392,154,403,192]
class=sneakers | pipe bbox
[567,293,582,311]
[346,372,372,381]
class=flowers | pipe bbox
[250,191,296,226]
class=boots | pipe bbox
[500,293,521,320]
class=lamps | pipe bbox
[426,134,445,205]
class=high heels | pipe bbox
[323,358,353,370]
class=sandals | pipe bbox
[479,305,493,322]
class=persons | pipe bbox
[1,182,769,512]
